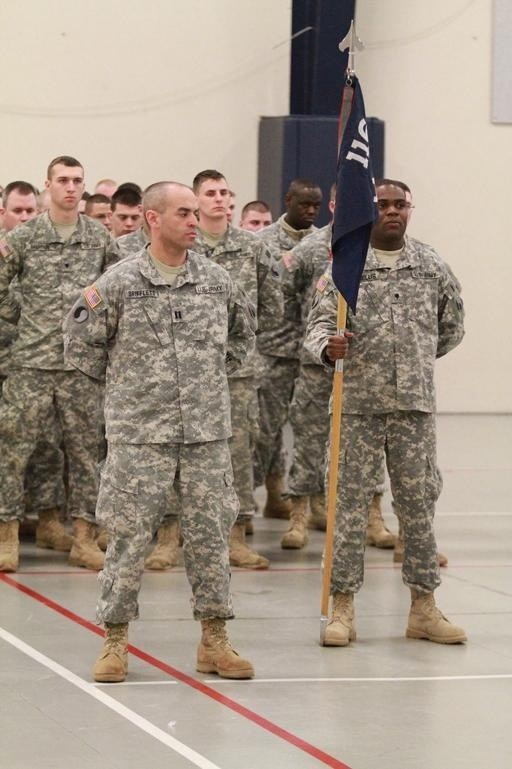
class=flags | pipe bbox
[331,76,379,317]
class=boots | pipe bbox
[241,518,254,534]
[68,518,107,571]
[35,511,75,550]
[308,499,328,531]
[393,515,448,566]
[196,619,254,679]
[144,519,180,570]
[0,519,19,572]
[281,496,311,549]
[224,526,268,569]
[406,587,469,644]
[93,622,129,682]
[367,494,398,548]
[322,592,357,646]
[95,529,109,551]
[262,499,293,521]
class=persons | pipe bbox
[61,181,256,685]
[0,178,73,555]
[224,187,237,227]
[74,192,90,217]
[0,156,108,575]
[96,222,183,572]
[95,177,117,198]
[278,182,395,550]
[301,180,470,648]
[109,187,143,239]
[245,180,327,535]
[144,170,283,572]
[120,181,143,197]
[37,188,51,214]
[238,200,293,519]
[391,179,450,567]
[84,193,111,232]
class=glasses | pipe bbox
[406,205,416,210]
